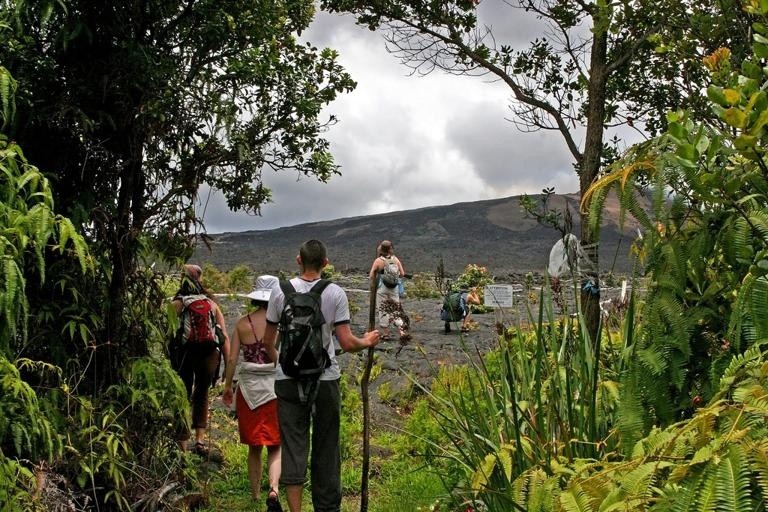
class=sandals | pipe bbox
[445,321,451,333]
[461,327,469,332]
[194,441,205,453]
[266,489,284,511]
[381,334,389,341]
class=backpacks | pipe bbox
[277,278,332,379]
[381,256,401,289]
[443,293,462,311]
[174,295,220,349]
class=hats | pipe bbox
[243,274,279,302]
[377,240,394,256]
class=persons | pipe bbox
[263,240,380,512]
[370,241,410,341]
[223,275,282,512]
[169,264,231,453]
[440,283,480,333]
